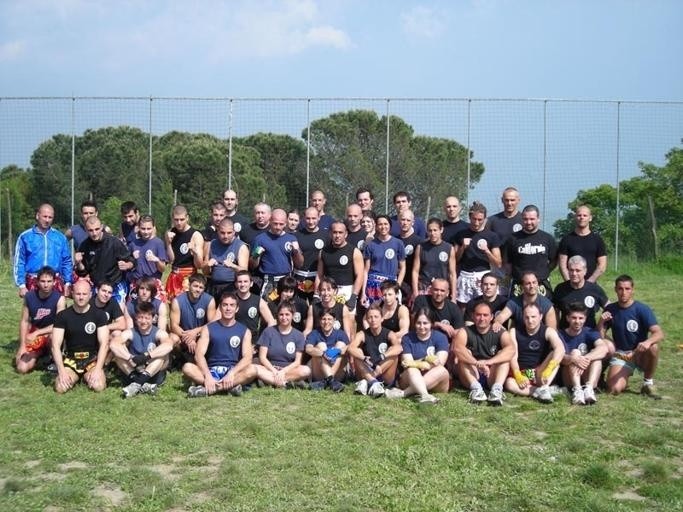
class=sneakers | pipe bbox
[532,385,554,404]
[549,383,569,396]
[418,393,438,405]
[469,384,488,402]
[48,362,58,371]
[486,383,505,407]
[120,380,250,400]
[572,384,586,405]
[286,378,345,392]
[584,383,597,404]
[352,377,406,400]
[641,384,662,400]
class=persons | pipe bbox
[558,304,608,405]
[182,292,255,397]
[305,308,349,390]
[452,301,516,405]
[16,186,611,374]
[600,273,663,396]
[257,301,311,388]
[385,307,450,403]
[111,302,173,398]
[51,280,110,393]
[347,306,402,397]
[504,302,565,402]
